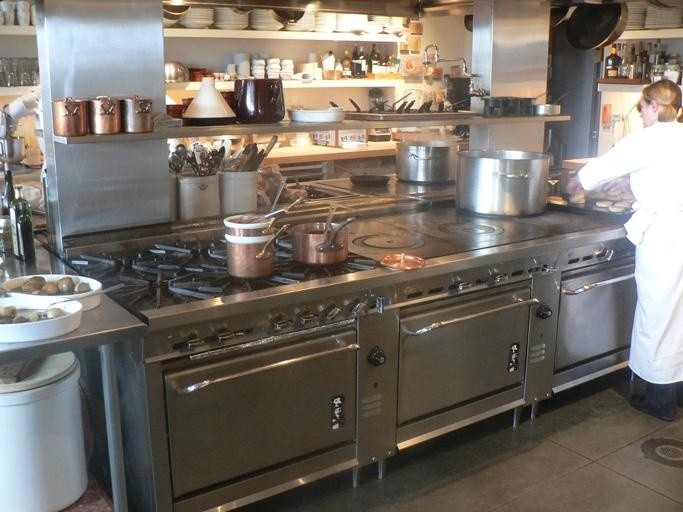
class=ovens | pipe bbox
[395,286,531,424]
[164,328,358,497]
[554,256,637,371]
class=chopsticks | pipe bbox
[291,218,349,264]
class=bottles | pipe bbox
[342,50,352,77]
[352,44,359,76]
[335,59,343,80]
[605,40,665,78]
[358,46,365,78]
[369,44,379,73]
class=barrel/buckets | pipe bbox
[0,356,89,512]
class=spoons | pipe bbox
[168,144,186,175]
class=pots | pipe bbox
[0,136,27,163]
[454,149,551,214]
[121,94,152,134]
[333,163,391,186]
[395,140,459,184]
[87,95,121,135]
[479,96,536,117]
[52,97,87,137]
[224,212,289,277]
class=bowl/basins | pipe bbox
[267,57,282,79]
[534,103,562,116]
[280,59,294,80]
[251,59,266,79]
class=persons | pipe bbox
[577,79,683,420]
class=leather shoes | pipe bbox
[629,393,677,422]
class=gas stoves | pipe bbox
[67,226,379,314]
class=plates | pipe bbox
[313,10,338,34]
[215,5,249,30]
[0,273,103,314]
[623,1,647,30]
[372,14,404,36]
[288,109,346,123]
[234,51,251,77]
[250,8,284,30]
[285,13,316,32]
[338,12,369,34]
[179,7,218,26]
[641,4,680,28]
[0,296,83,342]
